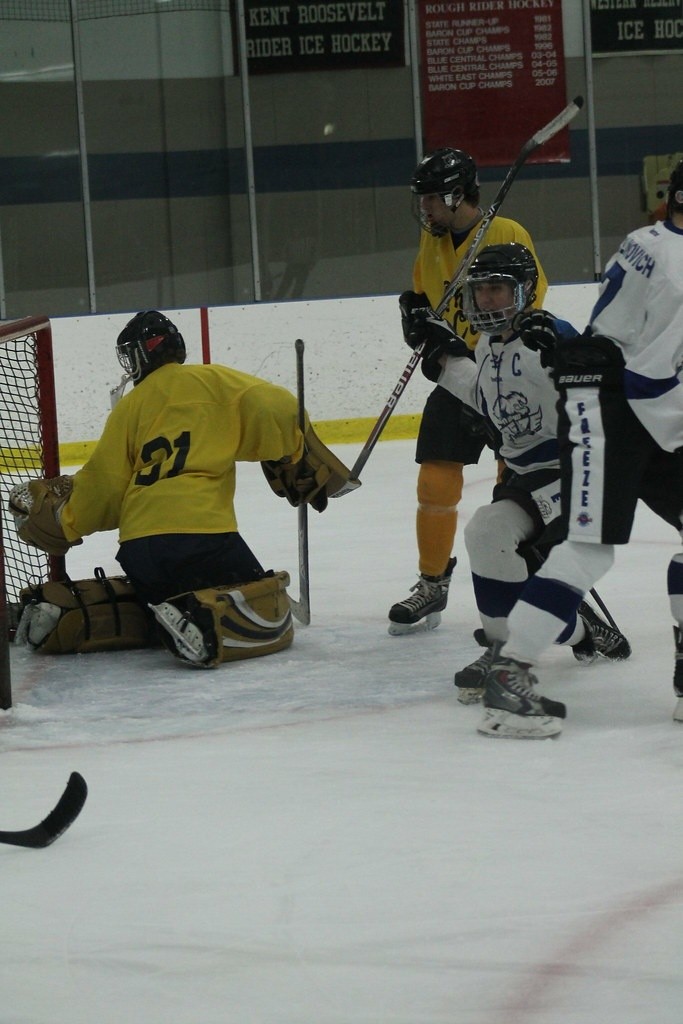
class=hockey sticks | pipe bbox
[348,98,583,482]
[282,338,310,624]
[0,772,88,849]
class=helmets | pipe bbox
[410,147,480,239]
[467,242,539,331]
[116,310,187,386]
[667,159,683,190]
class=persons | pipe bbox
[9,308,362,669]
[387,147,682,740]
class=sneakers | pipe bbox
[670,625,683,721]
[552,586,633,664]
[478,639,567,739]
[387,556,457,638]
[454,629,507,705]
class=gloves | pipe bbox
[519,312,560,369]
[398,290,469,383]
